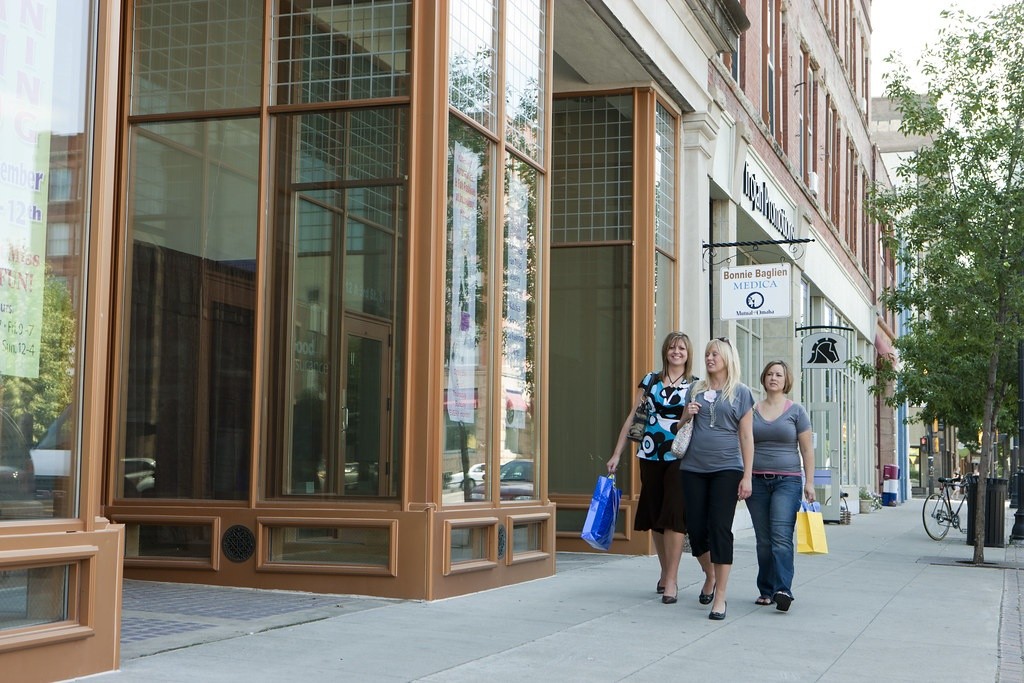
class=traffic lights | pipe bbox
[920,436,928,454]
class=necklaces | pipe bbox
[668,373,684,387]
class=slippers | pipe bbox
[754,594,774,604]
[773,591,791,611]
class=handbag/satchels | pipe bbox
[670,395,694,458]
[626,373,657,442]
[581,472,622,551]
[796,497,829,555]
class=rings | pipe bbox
[692,405,694,408]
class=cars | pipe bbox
[446,463,487,492]
[319,461,365,492]
[468,459,535,501]
[0,409,37,500]
[121,456,162,494]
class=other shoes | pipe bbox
[662,585,678,604]
[699,582,716,604]
[656,579,665,593]
[709,600,727,619]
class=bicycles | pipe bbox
[922,472,970,540]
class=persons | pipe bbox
[676,335,756,620]
[606,332,700,604]
[745,360,817,612]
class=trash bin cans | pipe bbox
[966,475,1009,548]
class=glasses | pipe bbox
[715,336,732,347]
[672,332,687,337]
